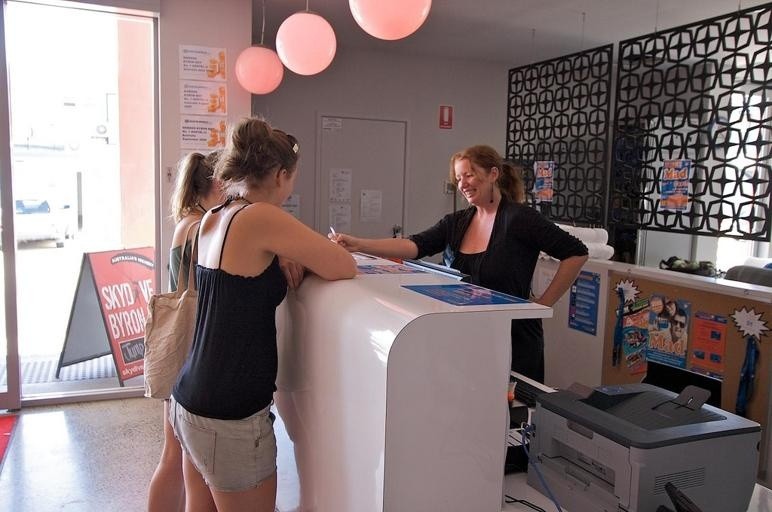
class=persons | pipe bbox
[658,308,687,349]
[145,145,236,512]
[166,117,359,511]
[326,142,590,385]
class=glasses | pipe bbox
[671,318,686,329]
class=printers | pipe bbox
[526,382,763,512]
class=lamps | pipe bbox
[234,0,339,96]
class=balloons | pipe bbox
[234,44,287,96]
[347,0,434,41]
[276,10,338,76]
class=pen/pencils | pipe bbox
[329,227,338,240]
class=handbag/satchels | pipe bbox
[143,289,198,401]
